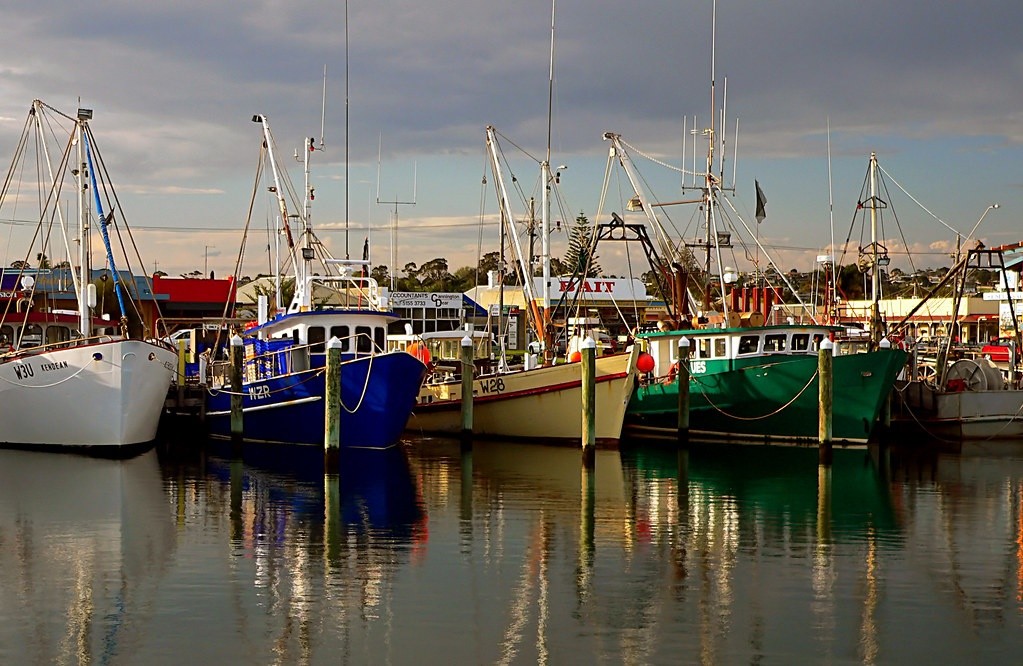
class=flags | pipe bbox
[755,180,768,224]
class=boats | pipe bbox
[191,442,909,562]
[600,1,1023,452]
[206,60,429,452]
[2,87,181,452]
[407,0,640,446]
